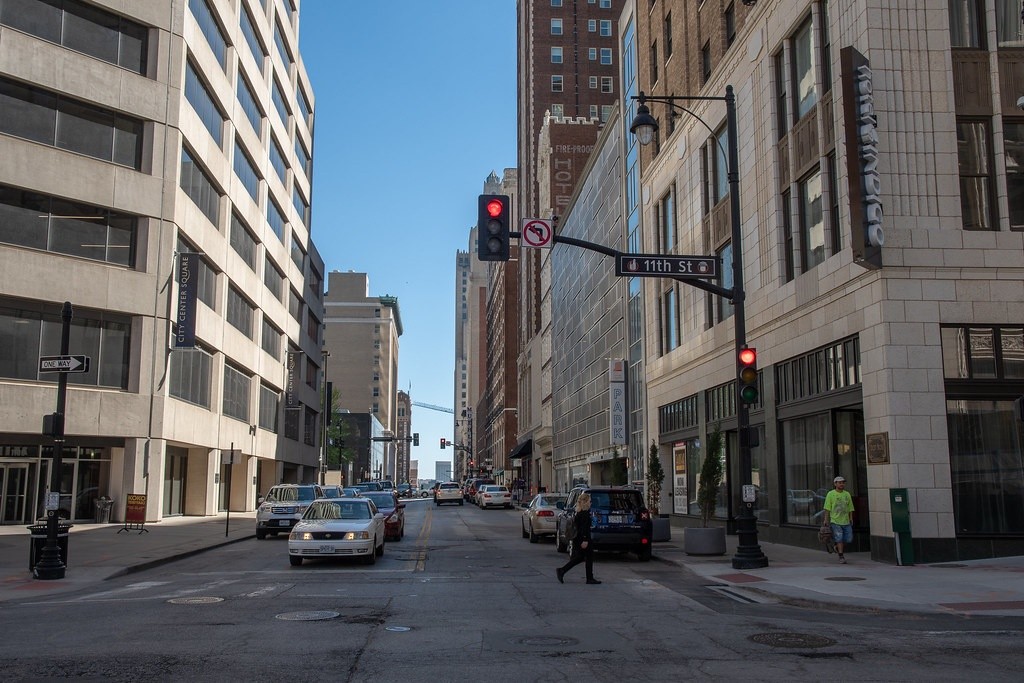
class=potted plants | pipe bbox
[646,438,671,542]
[684,420,727,555]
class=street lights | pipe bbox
[629,83,770,571]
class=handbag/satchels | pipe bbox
[818,526,832,544]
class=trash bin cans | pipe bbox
[95,499,113,524]
[26,524,73,572]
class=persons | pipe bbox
[556,494,602,584]
[504,477,526,502]
[823,477,854,564]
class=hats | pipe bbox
[834,477,847,483]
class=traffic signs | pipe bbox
[39,354,87,373]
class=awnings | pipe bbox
[494,470,503,477]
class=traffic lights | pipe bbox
[441,439,445,449]
[738,348,761,404]
[481,195,507,257]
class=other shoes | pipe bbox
[586,578,601,584]
[555,568,564,584]
[834,545,839,555]
[838,556,846,564]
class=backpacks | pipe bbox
[564,516,578,540]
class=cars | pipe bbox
[478,485,511,510]
[411,488,422,500]
[421,482,444,498]
[352,491,406,542]
[520,492,573,544]
[288,497,386,565]
[317,485,346,499]
[435,482,464,507]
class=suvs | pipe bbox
[556,483,654,561]
[396,483,413,498]
[343,480,395,499]
[461,477,497,507]
[256,482,328,541]
